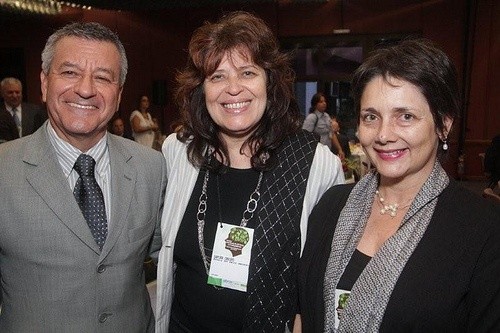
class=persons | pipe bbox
[1,22,169,333]
[294,40,500,333]
[129,95,158,149]
[155,13,345,333]
[109,116,137,143]
[0,77,49,143]
[302,93,345,161]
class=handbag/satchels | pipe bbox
[311,130,321,142]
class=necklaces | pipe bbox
[372,185,413,217]
[197,143,270,290]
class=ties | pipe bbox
[73,154,108,251]
[11,107,20,131]
